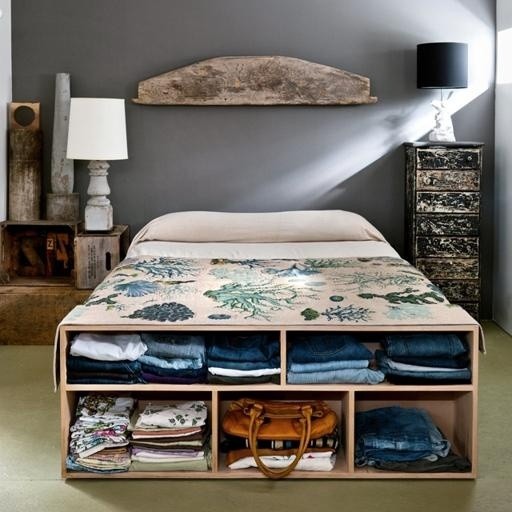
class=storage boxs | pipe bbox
[74,222,128,291]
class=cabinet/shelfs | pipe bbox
[2,218,78,292]
[401,141,483,320]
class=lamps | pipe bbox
[63,96,133,234]
[414,39,471,146]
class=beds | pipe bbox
[52,207,488,484]
